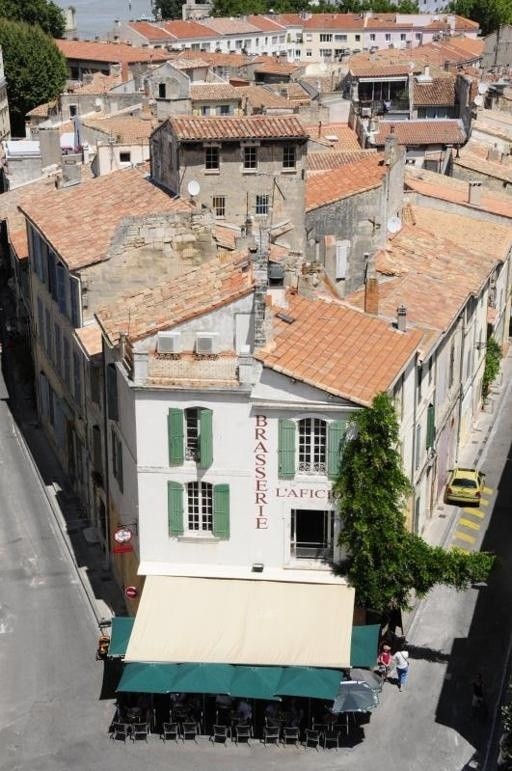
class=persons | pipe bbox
[388,643,410,694]
[379,645,392,684]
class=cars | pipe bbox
[444,466,488,508]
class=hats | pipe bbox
[384,645,391,650]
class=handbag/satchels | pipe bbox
[379,665,386,674]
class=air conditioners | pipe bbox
[155,330,220,358]
